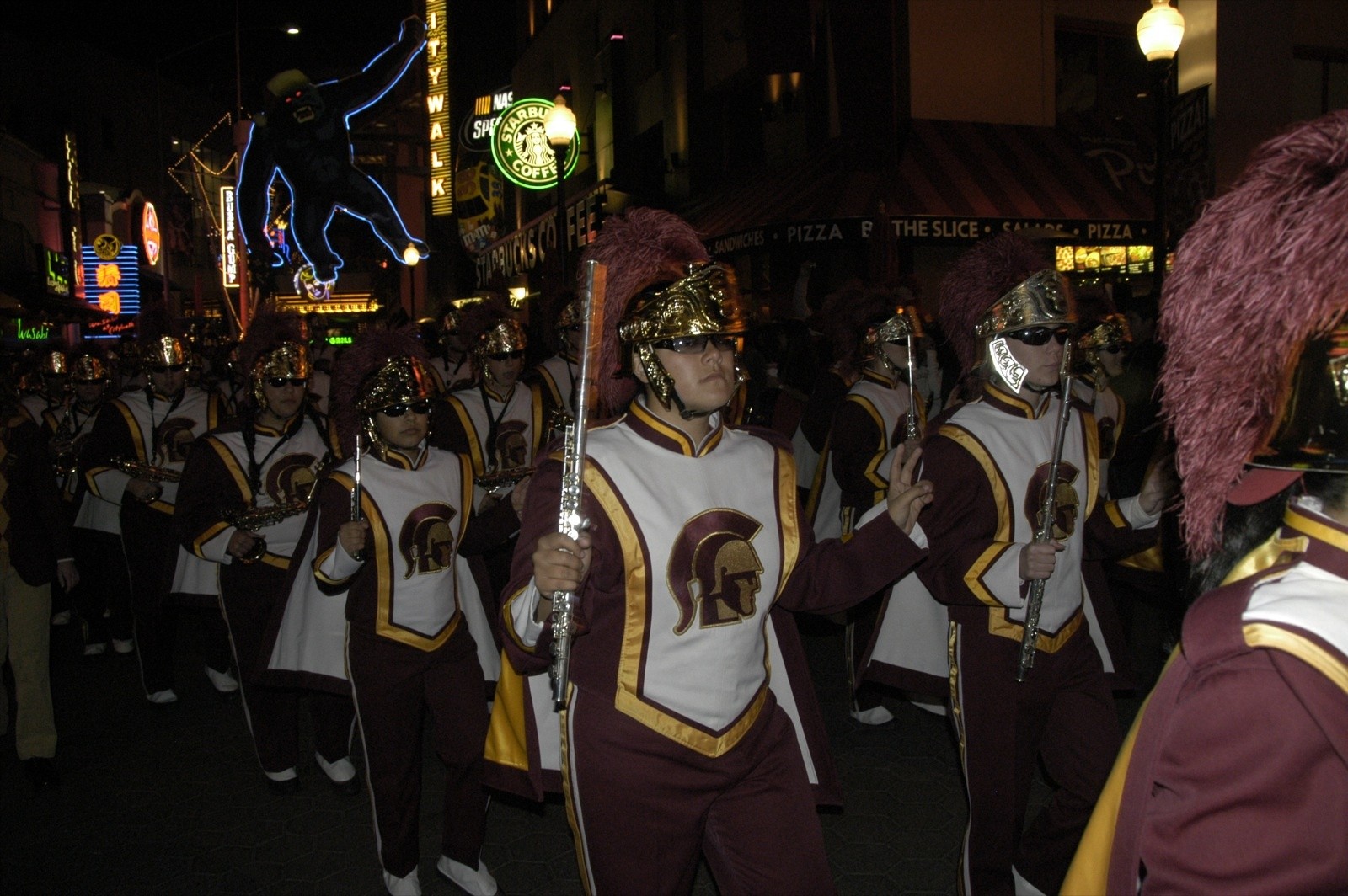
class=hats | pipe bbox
[6,111,1348,410]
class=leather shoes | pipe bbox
[22,756,57,792]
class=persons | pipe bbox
[0,116,1348,896]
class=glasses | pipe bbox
[569,324,581,333]
[489,350,524,361]
[372,399,433,418]
[151,363,185,374]
[886,338,908,346]
[1005,328,1068,346]
[653,333,738,352]
[262,377,307,389]
[1096,341,1131,355]
[75,376,105,386]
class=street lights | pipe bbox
[542,94,577,286]
[1134,0,1186,297]
[403,241,420,321]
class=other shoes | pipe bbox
[143,690,179,713]
[53,610,70,625]
[911,700,947,716]
[83,643,108,658]
[1011,865,1048,896]
[850,704,895,726]
[263,769,300,796]
[437,854,507,895]
[383,861,422,896]
[113,637,136,659]
[103,608,110,617]
[202,664,241,700]
[314,748,361,796]
